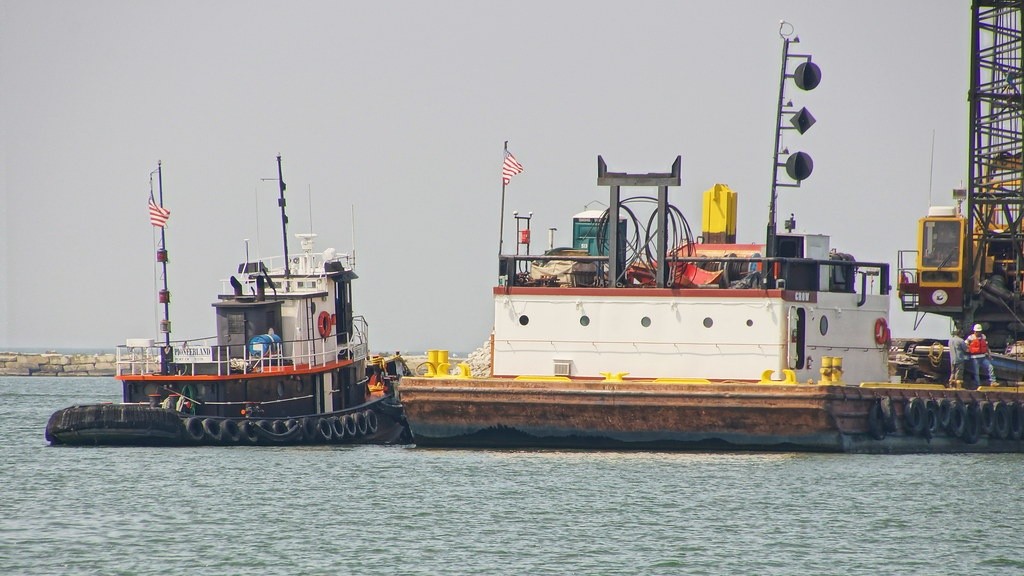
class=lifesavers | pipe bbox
[874,316,890,345]
[318,310,332,338]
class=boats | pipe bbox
[42,153,415,447]
[397,1,1024,452]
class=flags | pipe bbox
[148,197,170,227]
[500,148,523,185]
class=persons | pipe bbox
[946,329,970,390]
[964,323,1001,389]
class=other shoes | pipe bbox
[991,381,999,387]
[949,379,964,384]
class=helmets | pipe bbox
[973,324,983,332]
